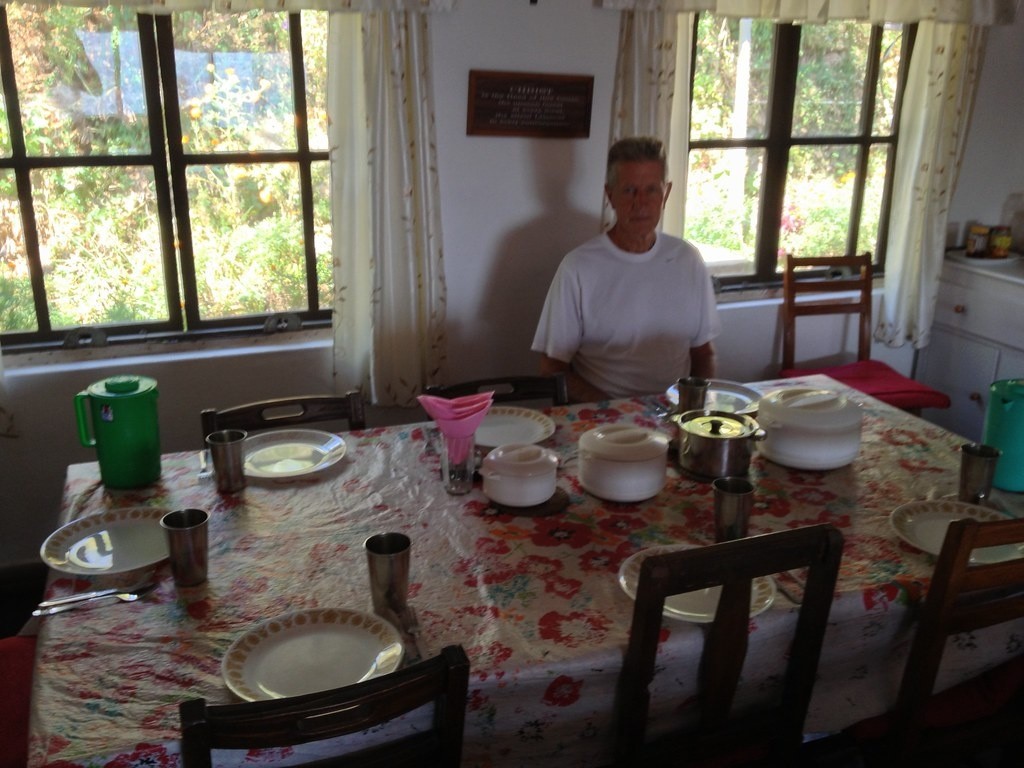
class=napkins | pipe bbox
[416,390,495,439]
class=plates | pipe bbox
[666,378,765,415]
[950,249,1019,268]
[244,429,346,478]
[40,505,179,574]
[220,606,405,701]
[619,501,1024,622]
[473,405,557,447]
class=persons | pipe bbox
[531,137,722,402]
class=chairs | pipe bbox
[783,250,950,418]
[613,523,846,768]
[200,389,367,450]
[427,370,578,425]
[180,644,471,768]
[886,517,1024,767]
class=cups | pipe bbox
[363,532,411,615]
[711,476,755,543]
[959,443,1002,503]
[678,376,711,413]
[159,507,212,587]
[440,433,475,494]
[205,429,248,493]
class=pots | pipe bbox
[479,422,669,507]
[670,410,768,479]
[753,387,864,471]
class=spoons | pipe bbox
[37,565,156,607]
[382,607,403,639]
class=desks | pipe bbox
[31,375,1024,768]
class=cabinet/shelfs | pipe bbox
[914,264,1024,442]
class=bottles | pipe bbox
[967,224,1012,258]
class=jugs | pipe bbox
[75,373,162,490]
[980,379,1024,493]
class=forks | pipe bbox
[197,449,212,479]
[403,604,430,662]
[32,582,160,615]
